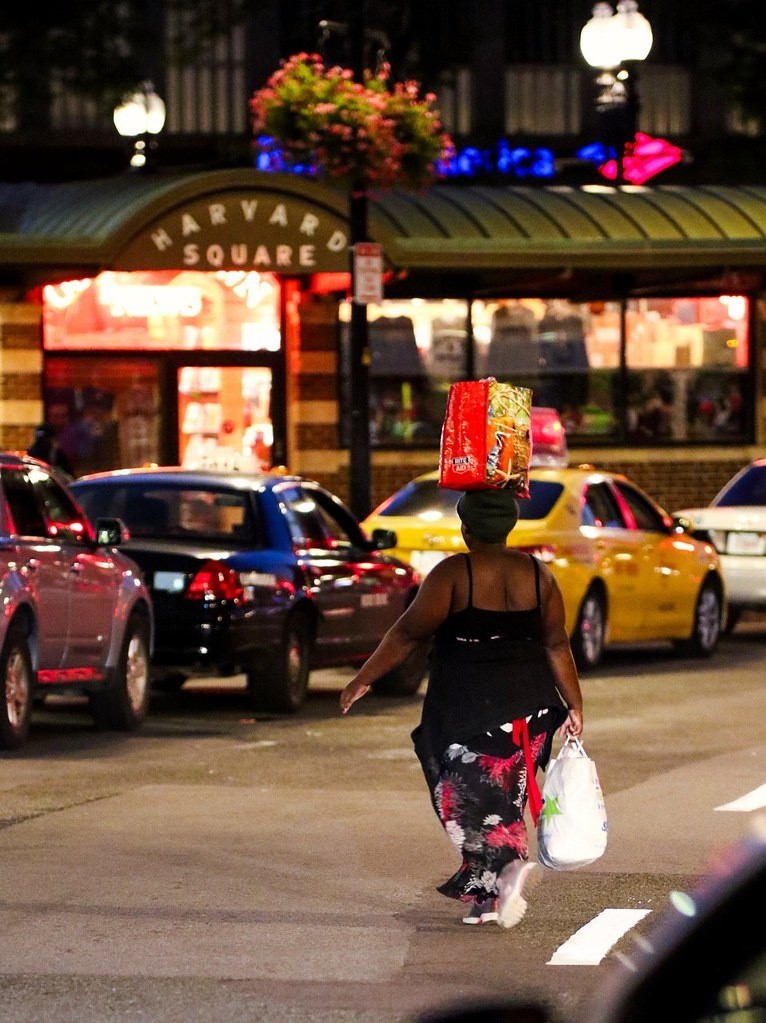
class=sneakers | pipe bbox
[463,897,499,924]
[496,858,542,928]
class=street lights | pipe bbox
[580,0,653,444]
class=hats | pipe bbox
[457,488,520,541]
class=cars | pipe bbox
[358,408,731,677]
[68,464,425,715]
[0,461,153,750]
[666,459,766,639]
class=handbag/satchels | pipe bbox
[537,736,607,871]
[437,377,532,500]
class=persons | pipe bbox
[30,402,110,478]
[339,487,585,929]
